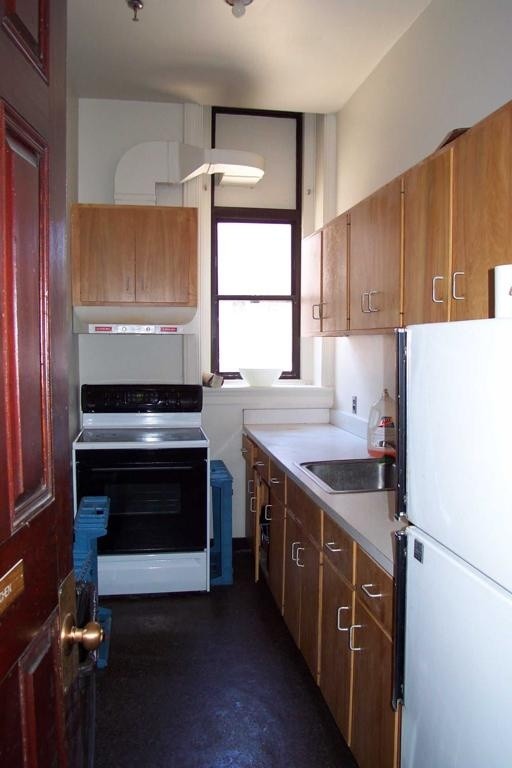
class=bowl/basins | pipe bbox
[239,368,283,386]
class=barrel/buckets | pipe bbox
[367,389,397,459]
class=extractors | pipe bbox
[73,305,198,335]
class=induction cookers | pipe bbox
[77,427,206,443]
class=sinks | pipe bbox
[294,455,398,494]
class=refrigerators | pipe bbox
[394,316,511,768]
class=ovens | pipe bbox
[72,454,210,557]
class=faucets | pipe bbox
[379,441,396,451]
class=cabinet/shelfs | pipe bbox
[346,174,403,336]
[71,205,201,324]
[270,459,287,617]
[243,430,260,587]
[320,509,355,751]
[255,445,270,588]
[301,210,351,336]
[284,470,324,687]
[349,540,398,766]
[403,99,512,323]
